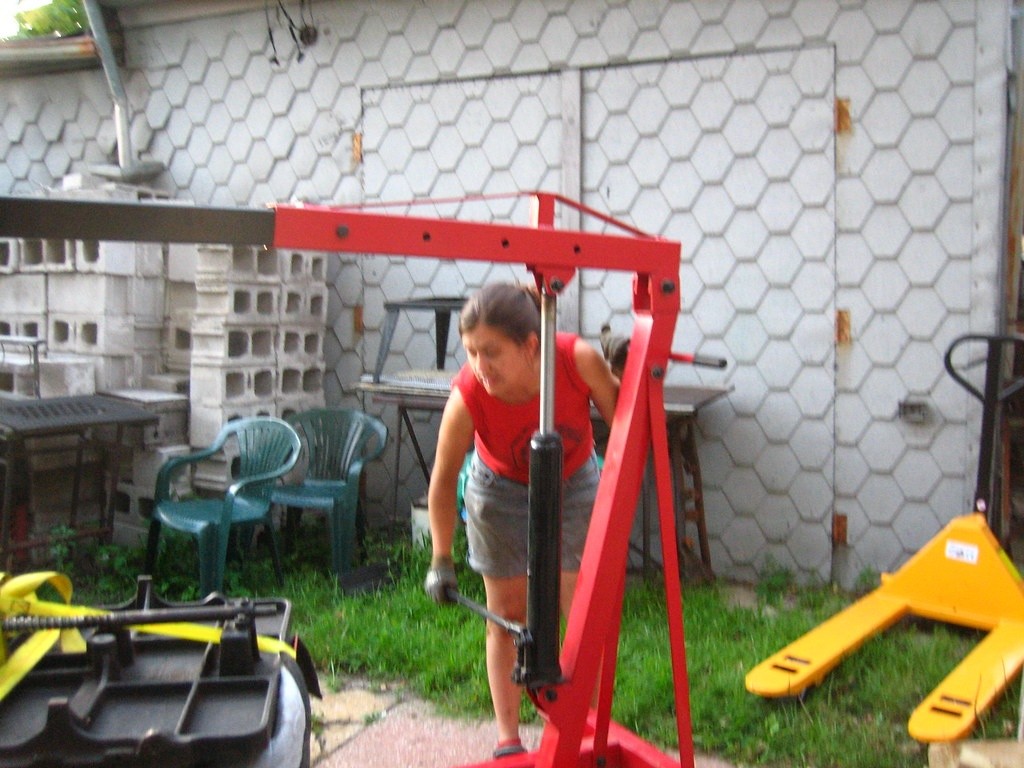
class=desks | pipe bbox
[373,385,726,590]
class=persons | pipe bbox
[423,283,621,758]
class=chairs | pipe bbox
[143,415,303,597]
[251,408,388,573]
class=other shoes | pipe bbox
[494,746,527,760]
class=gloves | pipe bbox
[424,555,458,604]
[599,324,631,375]
